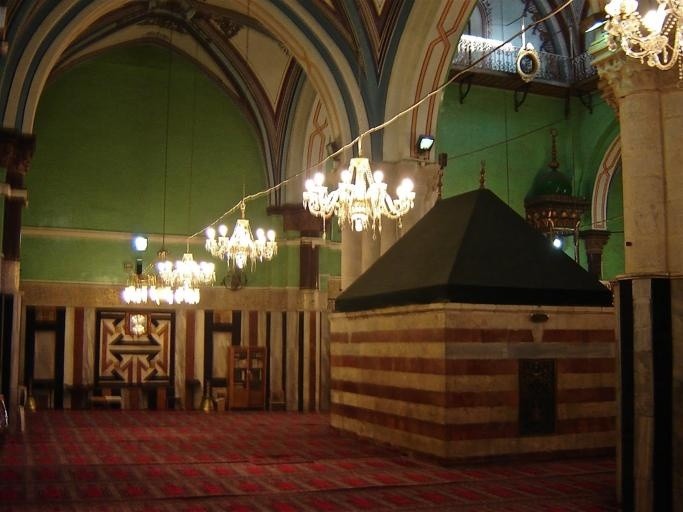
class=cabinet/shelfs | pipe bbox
[228,345,267,411]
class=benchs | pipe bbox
[88,396,124,410]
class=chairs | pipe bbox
[269,391,288,411]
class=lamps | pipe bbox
[603,0,683,80]
[154,246,220,286]
[416,134,435,153]
[201,216,281,276]
[299,138,416,244]
[123,260,203,306]
